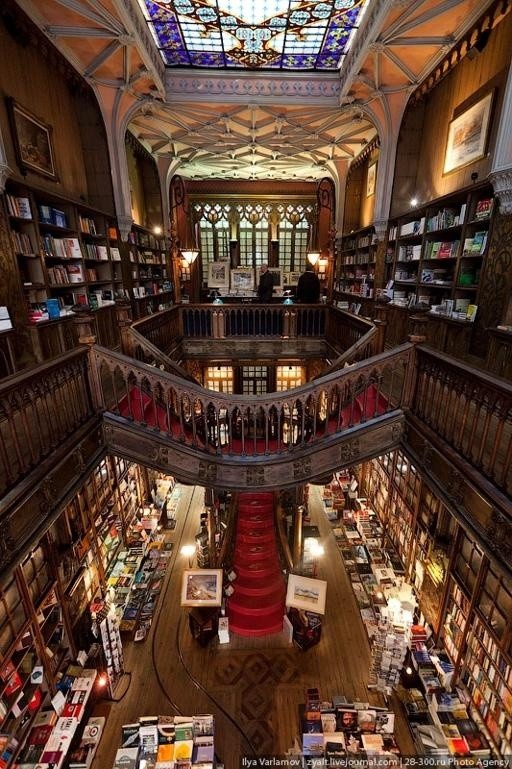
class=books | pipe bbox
[301,451,511,767]
[330,194,495,326]
[97,466,185,643]
[0,192,175,337]
[0,587,106,769]
[112,712,216,768]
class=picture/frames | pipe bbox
[207,261,230,288]
[442,86,496,177]
[366,159,378,199]
[285,573,328,616]
[6,94,61,184]
[180,567,224,608]
[230,268,284,291]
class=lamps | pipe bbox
[305,205,322,267]
[178,194,200,266]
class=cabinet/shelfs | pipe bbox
[188,608,220,648]
[287,607,323,652]
[349,448,512,768]
[331,179,512,423]
[0,174,175,421]
[0,462,167,769]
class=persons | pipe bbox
[255,263,273,305]
[295,265,322,303]
